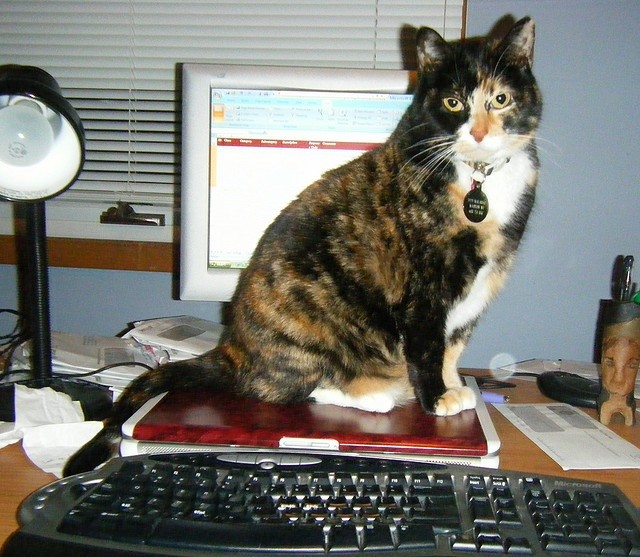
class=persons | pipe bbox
[597,333,639,429]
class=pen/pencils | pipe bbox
[620,255,634,301]
[610,255,624,300]
[480,392,510,403]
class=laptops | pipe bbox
[119,373,502,469]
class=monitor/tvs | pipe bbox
[179,61,419,302]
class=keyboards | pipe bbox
[15,450,639,556]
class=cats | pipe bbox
[62,13,544,480]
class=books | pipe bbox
[0,316,235,410]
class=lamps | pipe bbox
[0,63,113,424]
